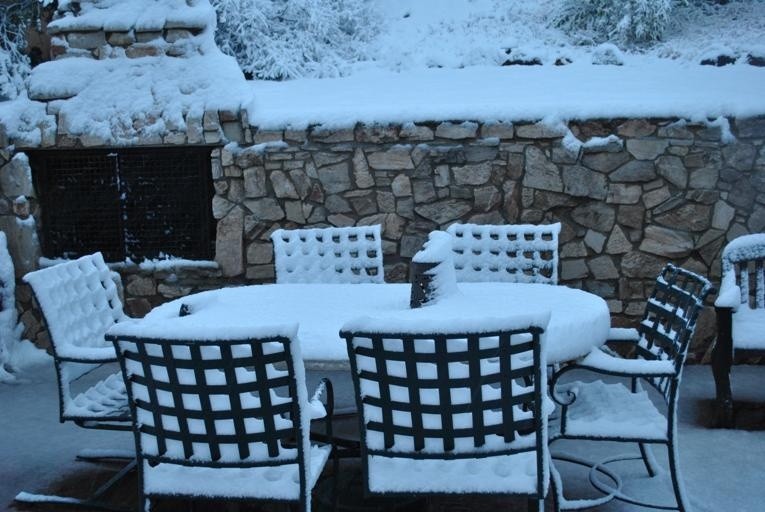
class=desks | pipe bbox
[137,281,623,511]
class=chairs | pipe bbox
[709,229,765,423]
[103,319,342,512]
[335,308,567,511]
[443,220,564,283]
[9,248,159,512]
[267,224,386,283]
[539,263,712,511]
